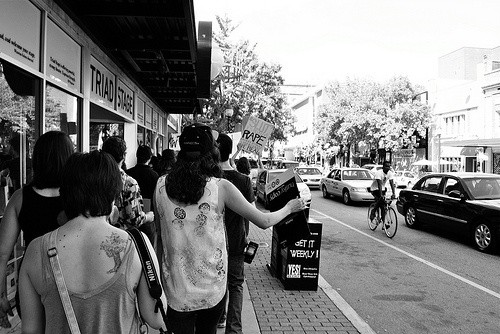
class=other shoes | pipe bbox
[370,209,375,221]
[216,315,227,328]
[382,225,391,230]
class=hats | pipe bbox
[179,123,214,161]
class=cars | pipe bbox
[361,164,394,172]
[294,166,331,190]
[259,156,300,168]
[388,171,420,189]
[320,167,392,206]
[255,169,312,210]
[395,171,500,253]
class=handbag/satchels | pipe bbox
[244,240,259,264]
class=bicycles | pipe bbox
[367,195,398,238]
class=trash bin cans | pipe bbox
[265,211,324,290]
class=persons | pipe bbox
[65,150,168,334]
[369,162,396,230]
[230,143,254,176]
[152,122,312,334]
[217,133,255,334]
[101,134,154,227]
[125,141,165,204]
[0,131,76,333]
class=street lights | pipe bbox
[226,106,233,133]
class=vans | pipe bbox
[235,157,264,190]
